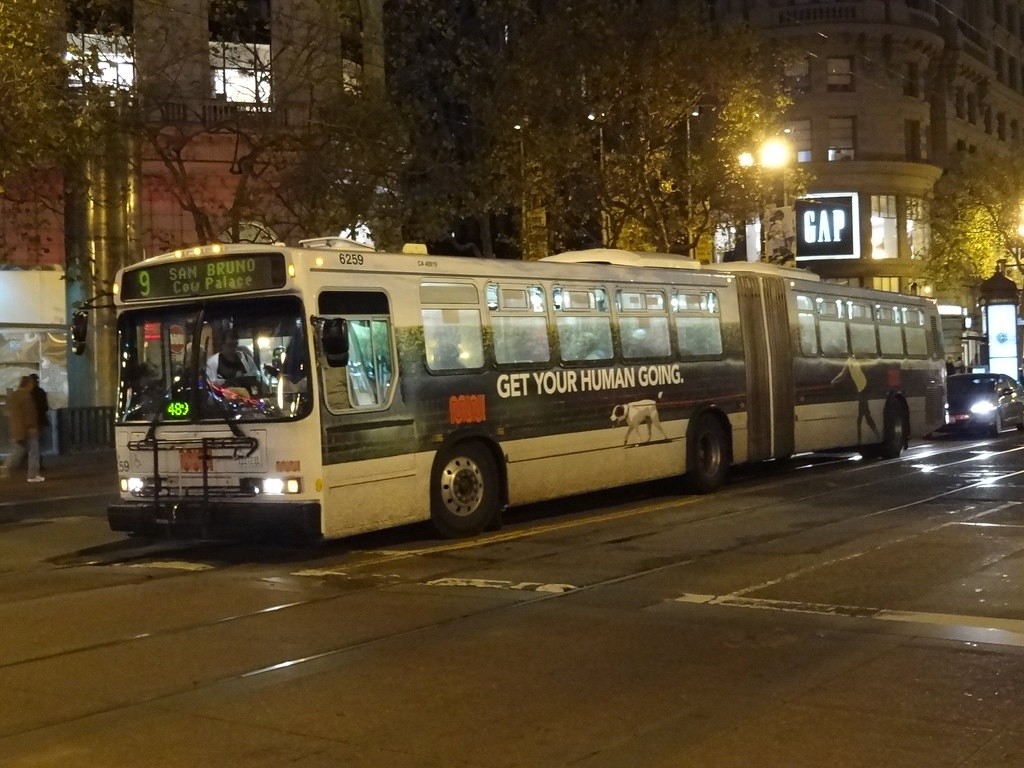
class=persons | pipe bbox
[946,356,962,377]
[170,320,374,411]
[554,288,563,309]
[7,374,47,482]
[530,288,543,312]
[971,353,978,366]
[576,332,605,360]
[627,328,656,357]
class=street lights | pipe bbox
[681,103,794,257]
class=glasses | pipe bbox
[224,342,238,348]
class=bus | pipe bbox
[67,236,949,548]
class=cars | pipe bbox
[923,373,1023,435]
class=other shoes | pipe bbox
[28,475,45,483]
